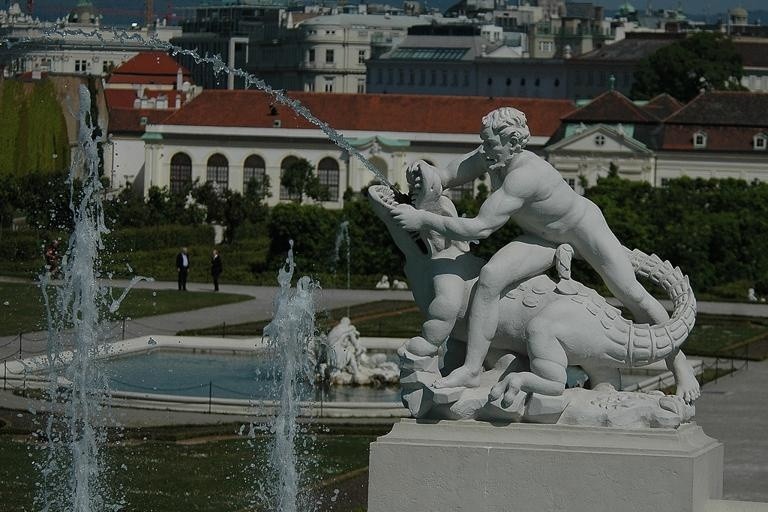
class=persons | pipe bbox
[210,250,222,292]
[176,247,191,292]
[390,103,703,405]
